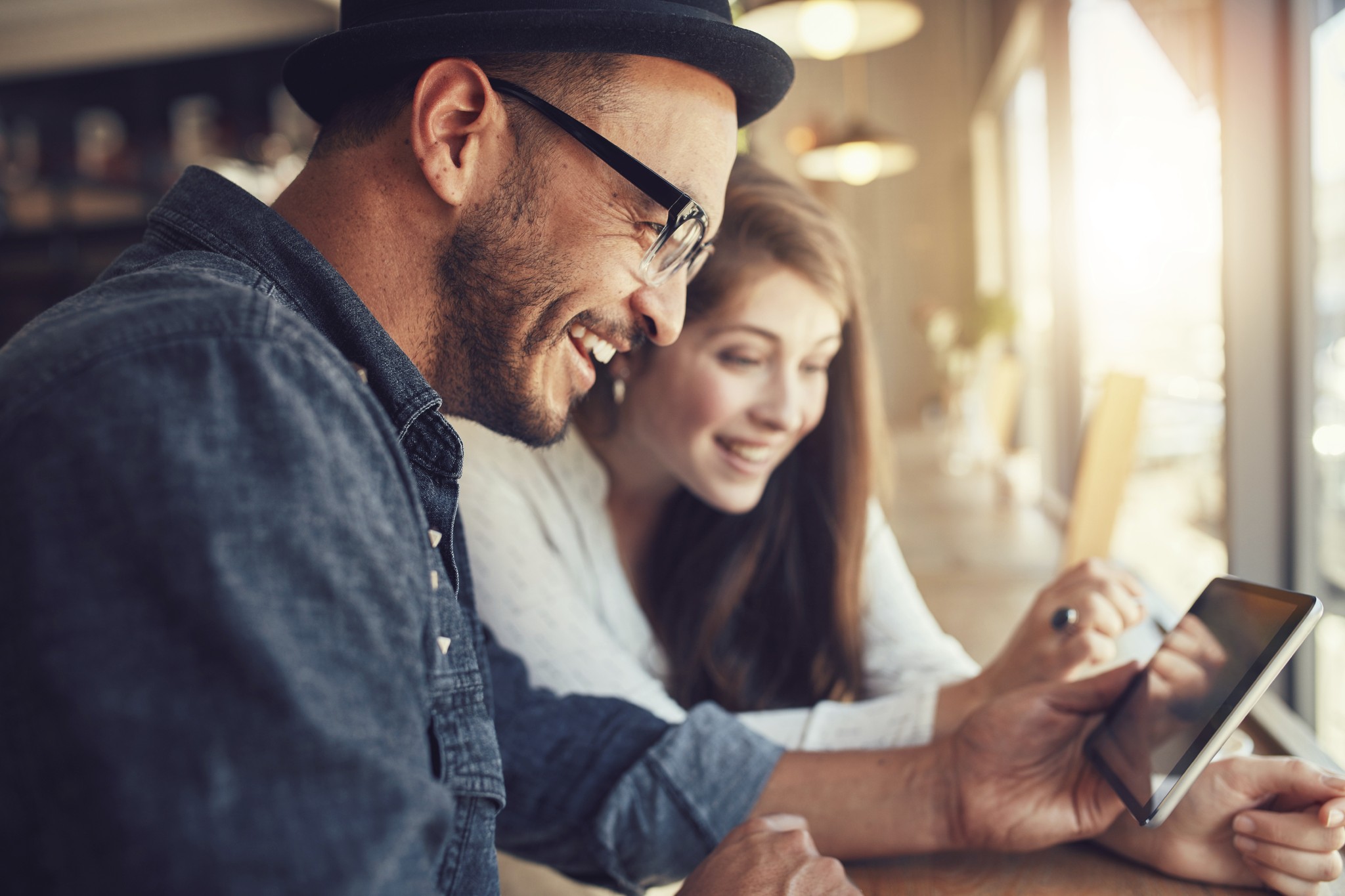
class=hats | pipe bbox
[284,1,795,130]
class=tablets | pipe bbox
[1080,576,1324,828]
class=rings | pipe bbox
[1051,607,1080,635]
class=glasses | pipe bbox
[483,74,714,286]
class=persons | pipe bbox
[2,2,1145,896]
[445,155,1345,896]
[1132,613,1228,718]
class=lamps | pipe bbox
[795,68,918,193]
[730,0,924,63]
